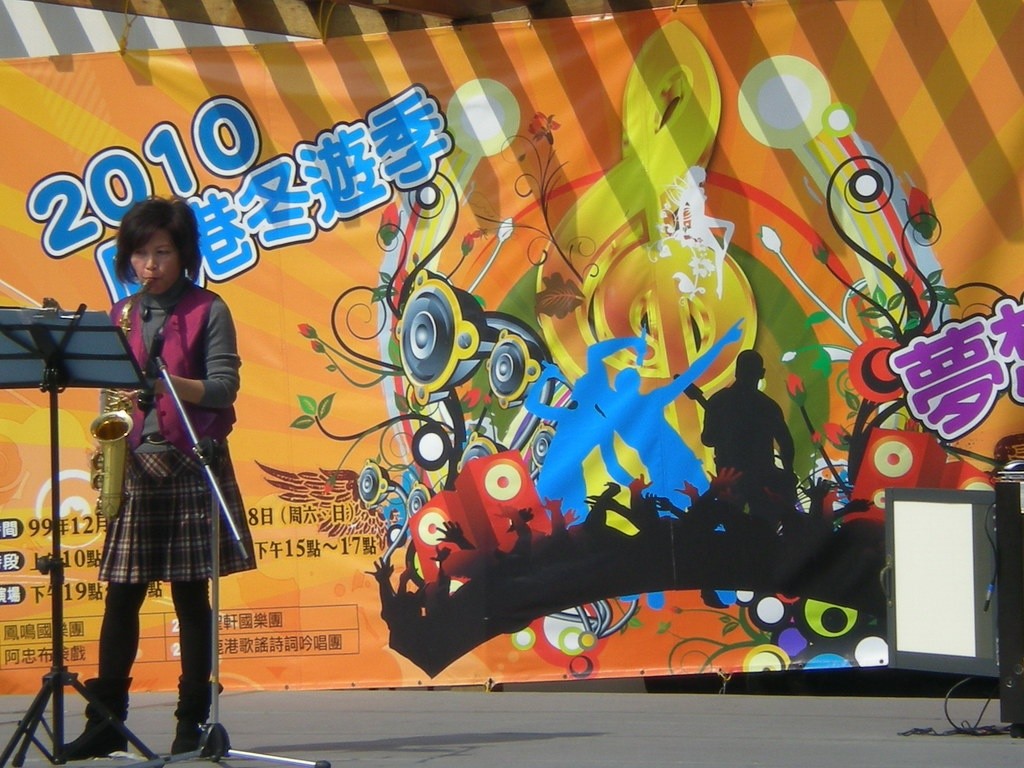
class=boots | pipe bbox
[171,675,224,756]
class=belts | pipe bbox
[142,432,168,444]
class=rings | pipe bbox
[135,390,138,394]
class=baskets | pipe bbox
[63,676,133,761]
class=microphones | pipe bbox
[136,332,165,412]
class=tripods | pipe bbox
[0,299,333,768]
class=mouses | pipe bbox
[1004,460,1024,472]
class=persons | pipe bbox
[60,194,257,761]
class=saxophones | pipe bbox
[88,277,158,520]
